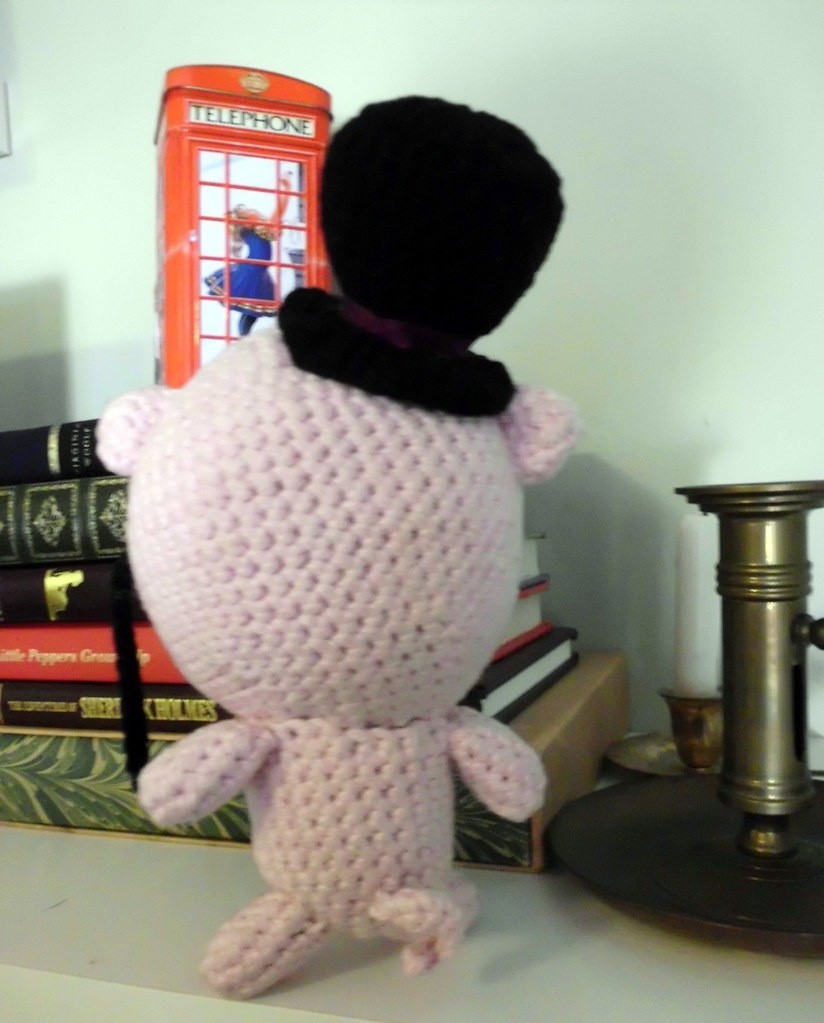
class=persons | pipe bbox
[205,167,294,337]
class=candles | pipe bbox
[676,508,721,696]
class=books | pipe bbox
[2,418,579,734]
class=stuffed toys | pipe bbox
[95,98,579,1002]
